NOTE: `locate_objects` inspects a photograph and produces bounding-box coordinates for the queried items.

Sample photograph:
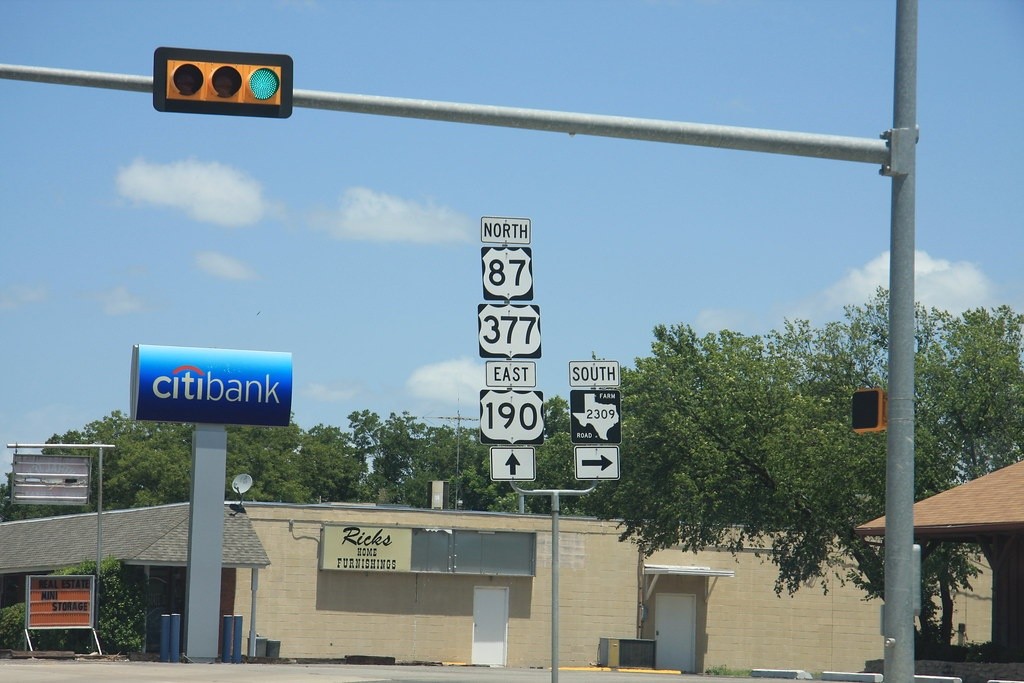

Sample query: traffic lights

[153,47,294,118]
[852,389,882,432]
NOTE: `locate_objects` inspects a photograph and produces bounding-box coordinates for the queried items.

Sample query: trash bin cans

[266,641,281,658]
[247,637,268,657]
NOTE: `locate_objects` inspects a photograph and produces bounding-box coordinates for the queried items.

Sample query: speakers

[852,389,887,436]
